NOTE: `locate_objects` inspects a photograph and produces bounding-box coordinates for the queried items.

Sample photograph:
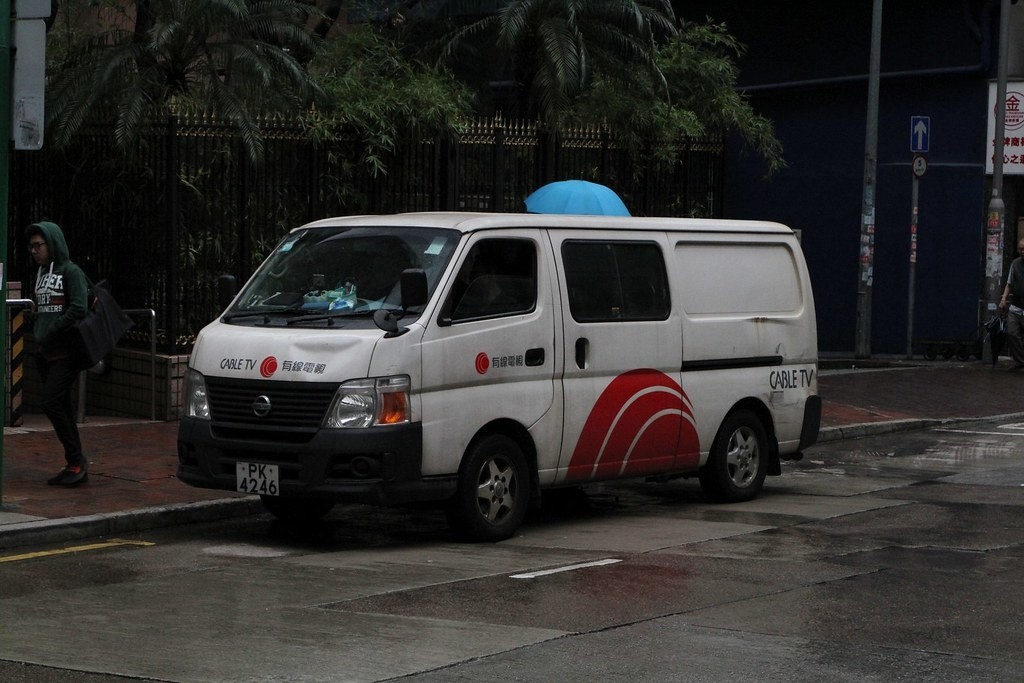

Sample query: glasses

[28,241,45,249]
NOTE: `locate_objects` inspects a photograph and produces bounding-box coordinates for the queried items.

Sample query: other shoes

[47,459,89,488]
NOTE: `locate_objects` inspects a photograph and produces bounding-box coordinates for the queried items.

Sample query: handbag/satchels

[63,263,135,368]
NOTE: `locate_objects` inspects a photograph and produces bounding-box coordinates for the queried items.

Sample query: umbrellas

[983,314,1006,335]
[525,178,632,217]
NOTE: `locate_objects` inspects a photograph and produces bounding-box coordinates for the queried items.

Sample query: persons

[998,239,1024,373]
[22,221,89,487]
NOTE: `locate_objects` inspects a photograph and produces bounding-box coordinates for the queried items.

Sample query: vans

[176,210,821,542]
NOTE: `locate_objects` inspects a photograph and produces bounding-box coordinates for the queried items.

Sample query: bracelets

[1001,299,1006,301]
